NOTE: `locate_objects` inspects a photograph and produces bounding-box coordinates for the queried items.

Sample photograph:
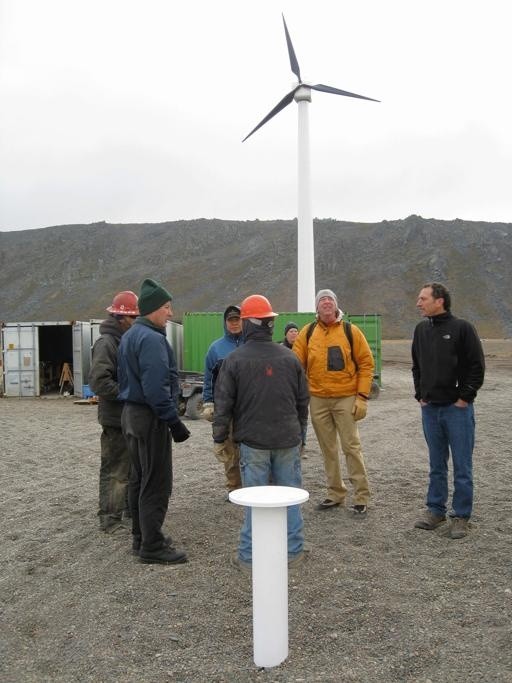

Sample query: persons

[115,279,191,565]
[411,282,485,539]
[212,295,310,574]
[276,322,309,458]
[88,290,141,537]
[201,305,244,503]
[292,289,375,519]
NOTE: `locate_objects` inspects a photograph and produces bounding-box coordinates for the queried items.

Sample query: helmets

[104,289,142,316]
[238,294,280,320]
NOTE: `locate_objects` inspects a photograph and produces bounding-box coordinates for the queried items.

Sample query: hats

[283,320,299,336]
[138,276,174,316]
[224,304,241,320]
[314,287,339,313]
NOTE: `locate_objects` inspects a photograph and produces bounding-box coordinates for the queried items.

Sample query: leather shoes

[224,489,236,503]
[138,541,189,566]
[131,531,174,557]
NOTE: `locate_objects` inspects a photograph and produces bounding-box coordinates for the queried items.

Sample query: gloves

[212,441,231,463]
[202,401,215,422]
[169,419,192,443]
[351,393,370,422]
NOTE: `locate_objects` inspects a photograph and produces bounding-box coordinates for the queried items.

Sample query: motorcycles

[175,370,205,420]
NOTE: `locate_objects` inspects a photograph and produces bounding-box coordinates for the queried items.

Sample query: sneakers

[314,496,344,511]
[352,502,368,520]
[228,548,253,575]
[412,506,448,531]
[286,542,314,570]
[447,514,471,539]
[103,521,132,536]
